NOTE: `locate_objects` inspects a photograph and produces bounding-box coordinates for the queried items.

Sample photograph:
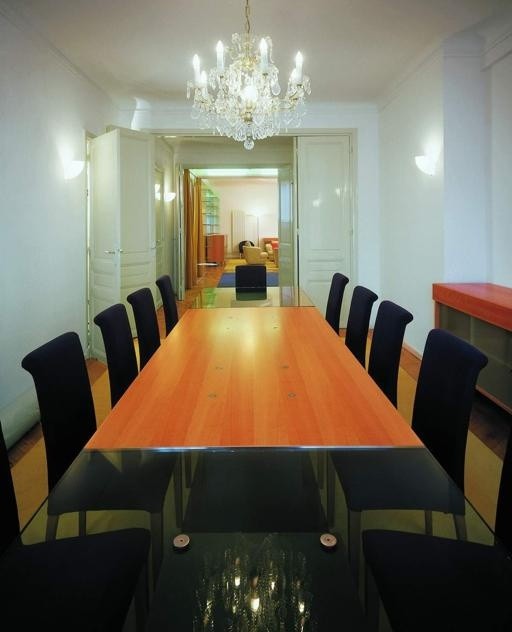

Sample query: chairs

[239,237,279,265]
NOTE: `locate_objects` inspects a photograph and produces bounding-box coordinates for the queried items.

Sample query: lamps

[178,0,313,152]
